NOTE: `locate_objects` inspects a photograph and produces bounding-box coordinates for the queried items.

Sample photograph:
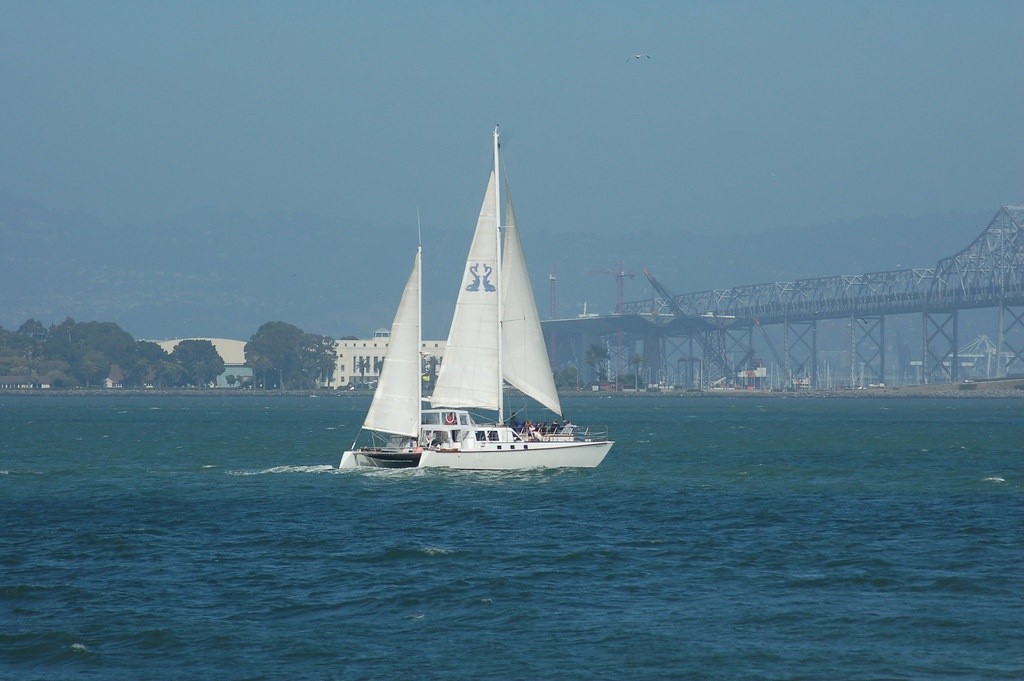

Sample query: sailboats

[337,121,617,473]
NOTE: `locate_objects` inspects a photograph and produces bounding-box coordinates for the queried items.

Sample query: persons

[510,413,569,433]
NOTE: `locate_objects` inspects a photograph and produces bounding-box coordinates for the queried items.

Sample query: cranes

[637,266,738,390]
[548,262,558,319]
[587,260,636,314]
